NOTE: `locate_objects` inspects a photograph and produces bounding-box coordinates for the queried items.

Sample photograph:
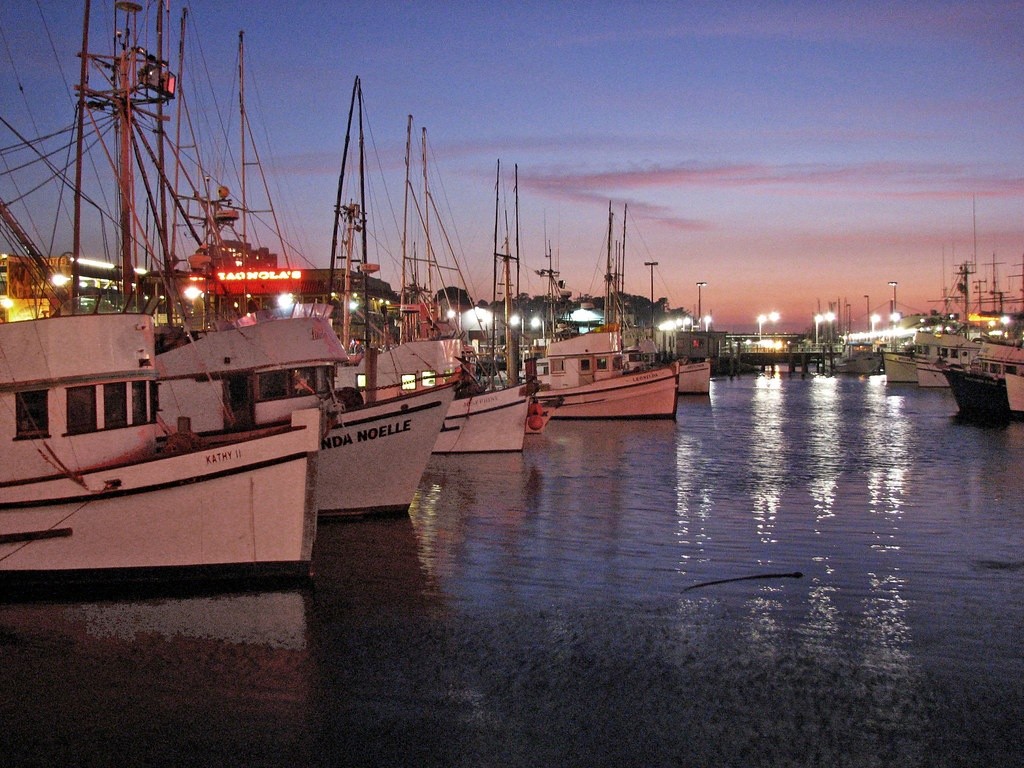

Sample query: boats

[1,1,710,571]
[834,195,1024,417]
